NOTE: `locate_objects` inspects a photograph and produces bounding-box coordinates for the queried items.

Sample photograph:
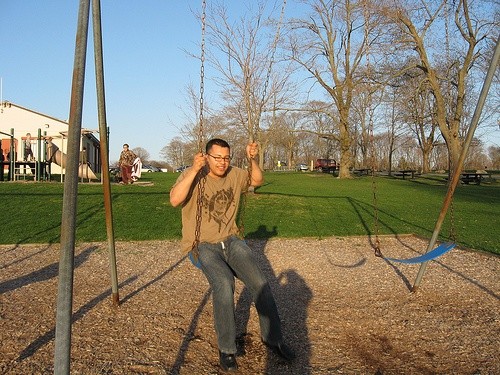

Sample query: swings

[362,0,457,266]
[189,0,287,269]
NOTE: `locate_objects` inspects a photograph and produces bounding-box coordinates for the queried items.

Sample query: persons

[117,144,139,184]
[171,139,295,371]
[24,133,35,162]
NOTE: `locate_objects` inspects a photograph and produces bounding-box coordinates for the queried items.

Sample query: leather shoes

[262,338,295,361]
[218,349,237,368]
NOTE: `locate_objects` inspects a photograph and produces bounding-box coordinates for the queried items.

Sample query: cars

[276,161,287,167]
[141,164,161,173]
[295,163,309,172]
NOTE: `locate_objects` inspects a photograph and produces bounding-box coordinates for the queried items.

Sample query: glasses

[206,153,232,161]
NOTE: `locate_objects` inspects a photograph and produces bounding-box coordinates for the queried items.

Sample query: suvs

[314,158,340,173]
